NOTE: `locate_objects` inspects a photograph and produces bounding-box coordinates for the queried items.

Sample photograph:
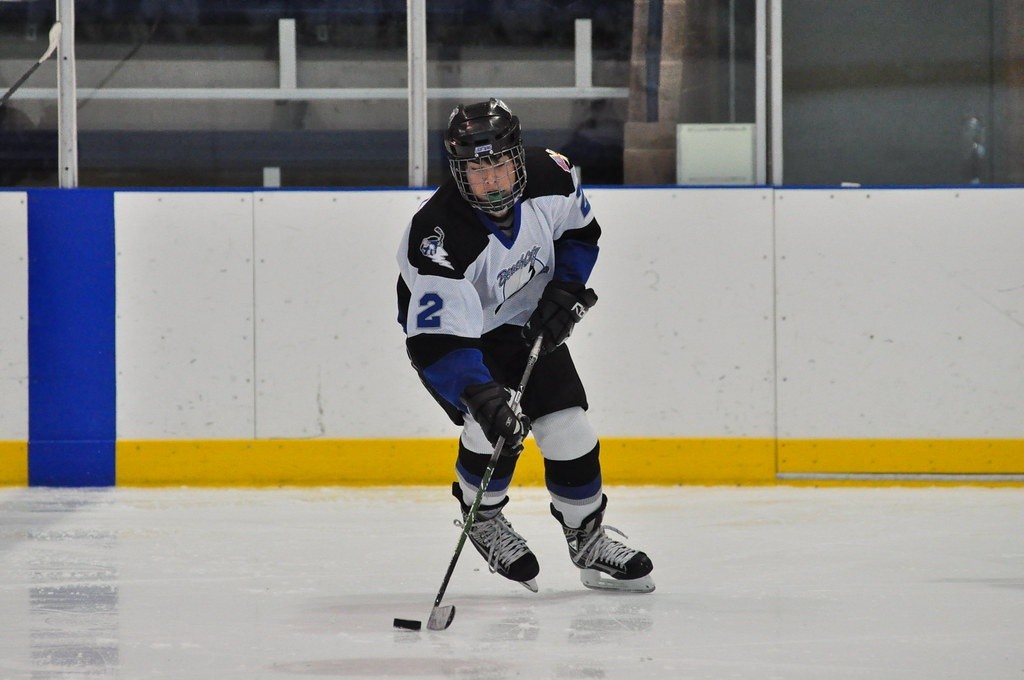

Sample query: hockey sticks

[426,335,546,632]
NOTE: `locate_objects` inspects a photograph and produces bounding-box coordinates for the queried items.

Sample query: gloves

[521,279,598,356]
[459,382,532,457]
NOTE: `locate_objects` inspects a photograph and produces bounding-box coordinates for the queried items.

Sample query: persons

[394,96,661,595]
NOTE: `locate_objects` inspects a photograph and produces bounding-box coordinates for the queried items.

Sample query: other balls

[393,617,421,630]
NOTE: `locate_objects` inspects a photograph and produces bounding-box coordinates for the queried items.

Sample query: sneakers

[452,482,540,593]
[550,494,656,593]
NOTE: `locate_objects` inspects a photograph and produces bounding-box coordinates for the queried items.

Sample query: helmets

[444,97,527,213]
